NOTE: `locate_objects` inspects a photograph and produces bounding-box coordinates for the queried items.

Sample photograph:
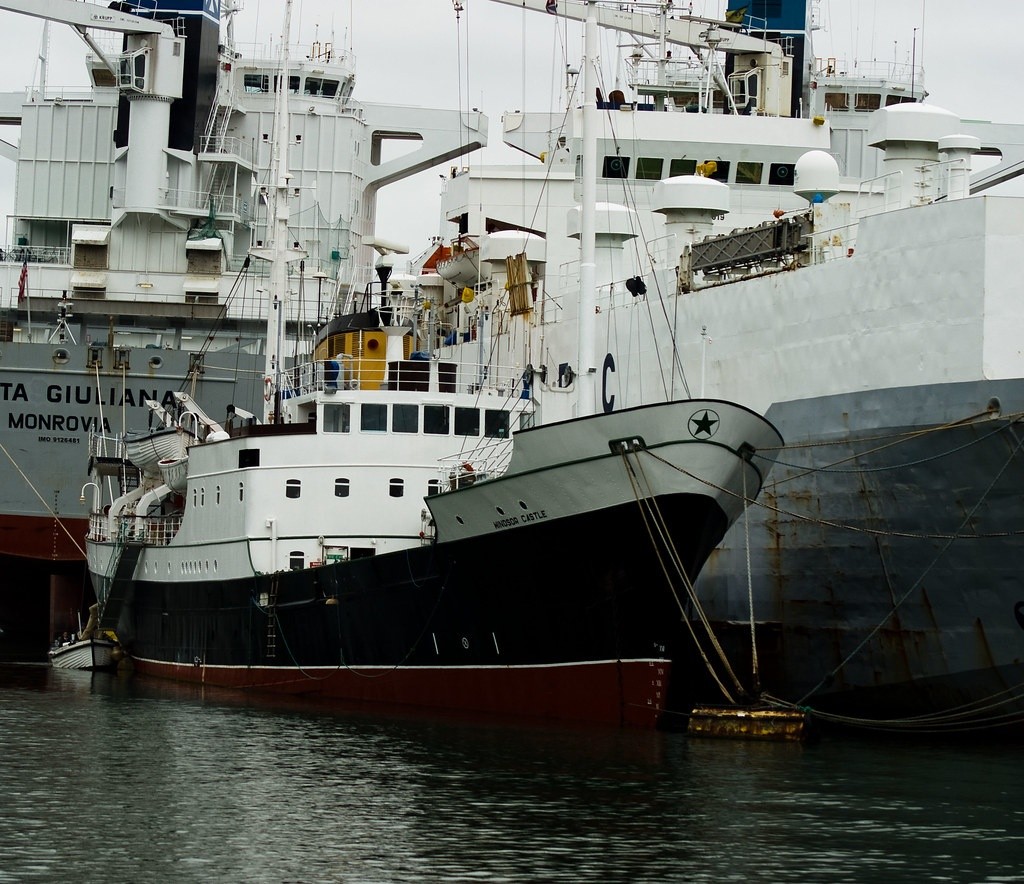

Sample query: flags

[18,252,28,303]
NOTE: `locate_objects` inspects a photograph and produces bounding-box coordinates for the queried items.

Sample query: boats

[2,1,1023,741]
[49,637,112,671]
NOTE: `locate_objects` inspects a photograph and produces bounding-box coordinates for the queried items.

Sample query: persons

[59,631,71,648]
